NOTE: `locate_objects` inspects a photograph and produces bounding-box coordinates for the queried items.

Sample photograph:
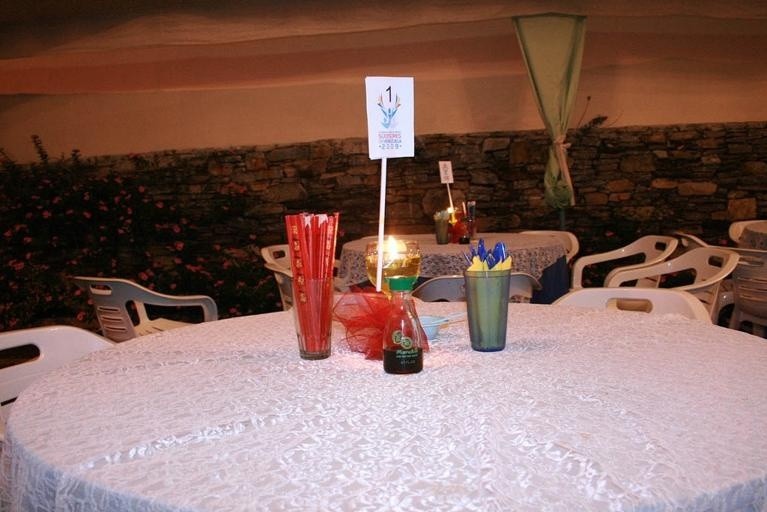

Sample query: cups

[289,276,335,361]
[463,268,512,353]
[435,222,449,244]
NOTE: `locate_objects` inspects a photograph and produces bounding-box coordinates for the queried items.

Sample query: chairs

[0,277,217,436]
[259,220,765,338]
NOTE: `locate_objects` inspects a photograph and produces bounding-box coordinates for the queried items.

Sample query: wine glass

[363,240,422,300]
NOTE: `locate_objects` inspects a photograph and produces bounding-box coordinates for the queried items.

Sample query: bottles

[382,276,423,375]
